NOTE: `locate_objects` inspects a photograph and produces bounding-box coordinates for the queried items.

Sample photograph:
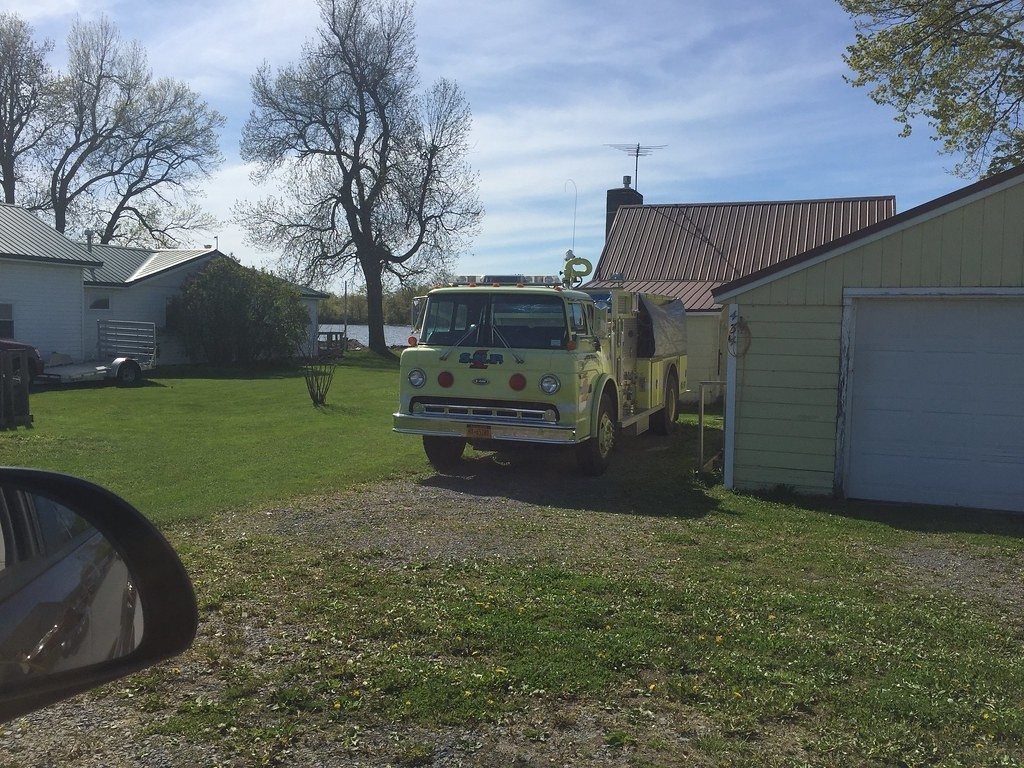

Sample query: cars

[0,340,44,392]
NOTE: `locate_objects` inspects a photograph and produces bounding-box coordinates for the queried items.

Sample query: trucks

[393,257,687,477]
